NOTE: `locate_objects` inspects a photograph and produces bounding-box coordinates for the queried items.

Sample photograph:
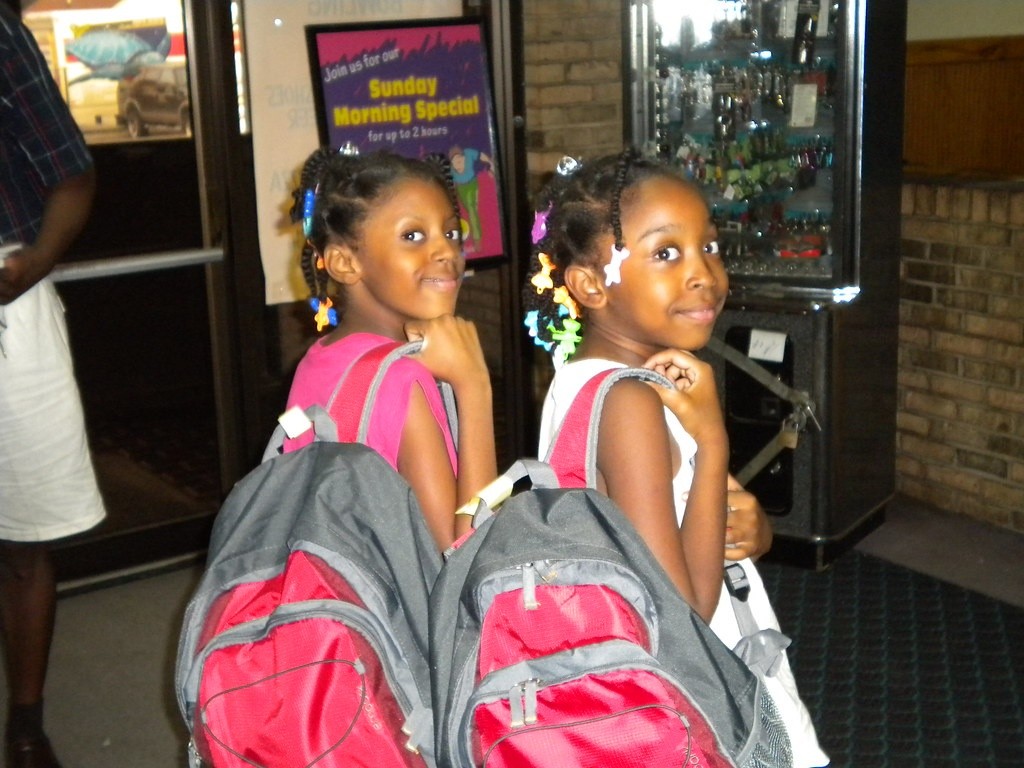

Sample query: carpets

[754,548,1024,768]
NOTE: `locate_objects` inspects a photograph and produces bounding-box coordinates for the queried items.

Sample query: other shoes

[4,728,61,768]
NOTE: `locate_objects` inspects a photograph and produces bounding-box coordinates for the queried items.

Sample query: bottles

[646,1,841,271]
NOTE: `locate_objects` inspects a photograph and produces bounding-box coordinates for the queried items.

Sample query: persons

[0,14,113,767]
[522,152,830,768]
[284,142,494,563]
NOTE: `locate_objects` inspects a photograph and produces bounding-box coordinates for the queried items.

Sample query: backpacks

[431,369,808,768]
[172,340,458,768]
